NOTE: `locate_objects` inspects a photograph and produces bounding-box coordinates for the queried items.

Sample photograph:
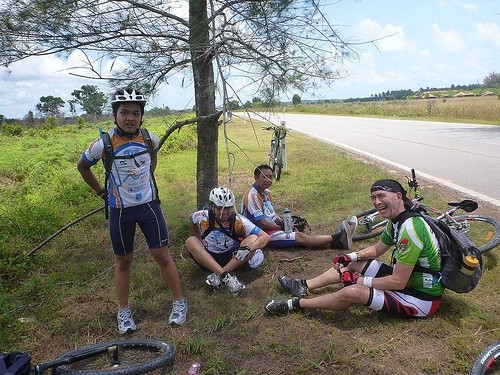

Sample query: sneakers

[347,215,358,236]
[265,296,301,317]
[339,221,353,250]
[168,298,189,326]
[222,272,246,296]
[278,275,310,299]
[117,307,137,335]
[206,272,223,291]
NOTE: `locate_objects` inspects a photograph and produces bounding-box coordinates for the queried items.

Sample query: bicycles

[341,168,500,257]
[262,123,292,183]
[1,338,201,375]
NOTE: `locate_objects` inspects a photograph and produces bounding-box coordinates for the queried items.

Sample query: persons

[265,179,445,317]
[240,165,357,250]
[77,89,187,335]
[186,186,268,296]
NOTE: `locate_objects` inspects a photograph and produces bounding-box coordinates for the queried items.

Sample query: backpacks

[281,215,311,234]
[390,208,483,293]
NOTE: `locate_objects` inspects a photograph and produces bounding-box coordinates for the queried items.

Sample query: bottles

[283,208,293,233]
[455,255,479,289]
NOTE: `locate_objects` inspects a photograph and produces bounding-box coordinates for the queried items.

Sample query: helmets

[208,186,235,207]
[111,87,147,115]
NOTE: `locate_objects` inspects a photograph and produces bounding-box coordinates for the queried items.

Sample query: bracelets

[95,188,108,199]
[363,276,373,287]
[346,251,362,262]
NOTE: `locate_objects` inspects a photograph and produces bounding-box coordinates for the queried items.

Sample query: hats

[372,179,407,199]
